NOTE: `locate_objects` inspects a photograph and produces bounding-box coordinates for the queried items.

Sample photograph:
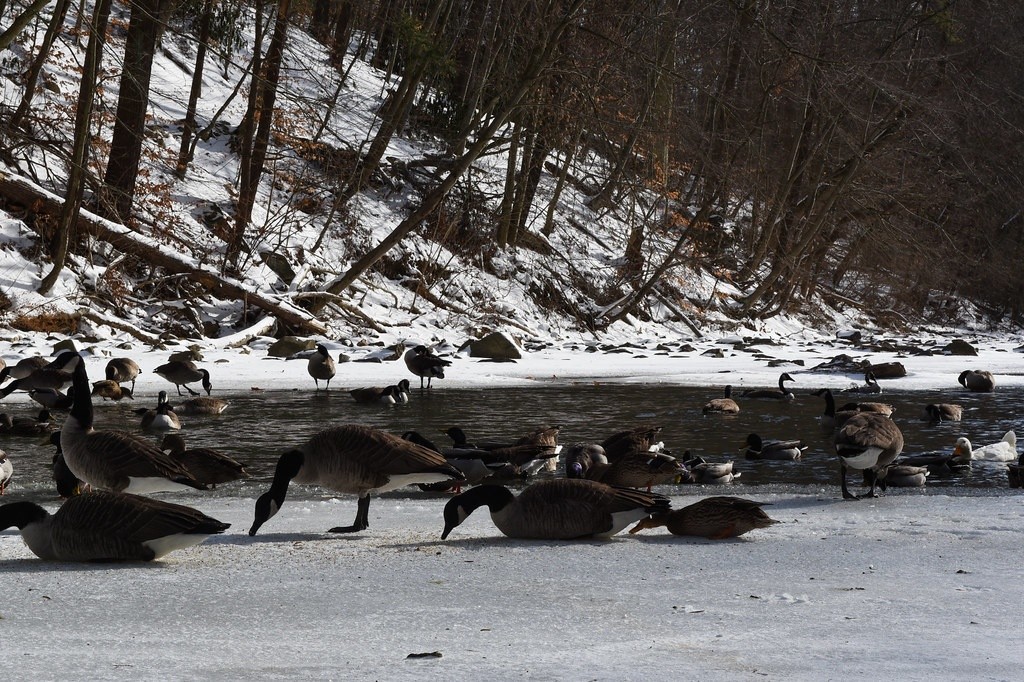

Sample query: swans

[953,429,1018,462]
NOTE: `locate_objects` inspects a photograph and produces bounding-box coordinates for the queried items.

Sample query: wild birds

[0,343,963,567]
[958,370,995,391]
